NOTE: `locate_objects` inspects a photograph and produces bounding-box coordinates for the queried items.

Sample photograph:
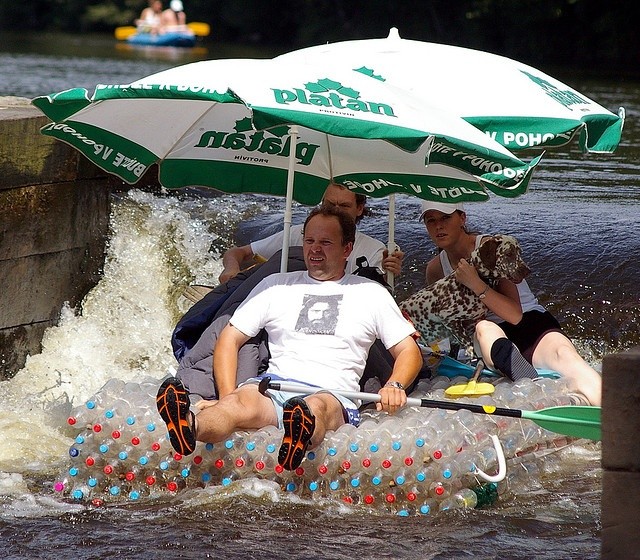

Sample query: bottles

[80,364,584,517]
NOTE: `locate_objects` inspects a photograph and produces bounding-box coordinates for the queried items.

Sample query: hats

[419,199,466,222]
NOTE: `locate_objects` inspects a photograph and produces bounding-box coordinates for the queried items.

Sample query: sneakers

[491,338,539,384]
[156,377,196,456]
[278,396,315,471]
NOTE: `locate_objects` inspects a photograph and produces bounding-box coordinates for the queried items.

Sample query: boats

[126,26,198,45]
[115,23,211,40]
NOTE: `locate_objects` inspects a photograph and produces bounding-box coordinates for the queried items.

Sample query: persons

[133,0,162,34]
[157,203,423,472]
[160,0,186,34]
[418,200,602,408]
[219,182,404,284]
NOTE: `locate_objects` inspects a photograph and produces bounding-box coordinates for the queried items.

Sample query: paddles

[258,377,601,439]
[446,357,494,396]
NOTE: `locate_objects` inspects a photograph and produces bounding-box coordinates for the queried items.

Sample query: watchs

[479,286,491,300]
[383,381,405,390]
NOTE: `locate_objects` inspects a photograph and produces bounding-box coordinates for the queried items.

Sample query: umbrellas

[271,27,620,295]
[29,58,546,273]
